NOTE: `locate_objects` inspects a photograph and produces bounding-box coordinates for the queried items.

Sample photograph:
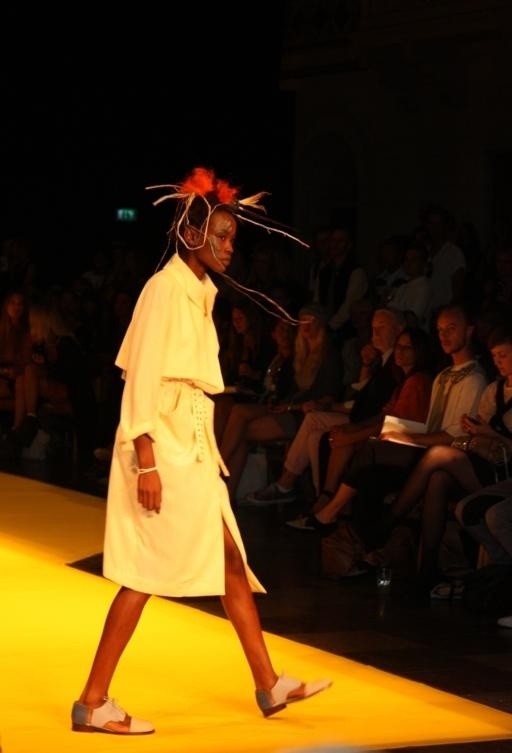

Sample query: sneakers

[247,482,295,504]
[285,515,338,533]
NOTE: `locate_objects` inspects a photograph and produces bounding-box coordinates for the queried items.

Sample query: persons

[67,188,337,738]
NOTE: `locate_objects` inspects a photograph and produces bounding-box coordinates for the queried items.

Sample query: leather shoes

[255,677,333,718]
[72,699,155,735]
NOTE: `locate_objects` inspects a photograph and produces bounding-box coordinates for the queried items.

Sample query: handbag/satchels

[451,435,510,466]
